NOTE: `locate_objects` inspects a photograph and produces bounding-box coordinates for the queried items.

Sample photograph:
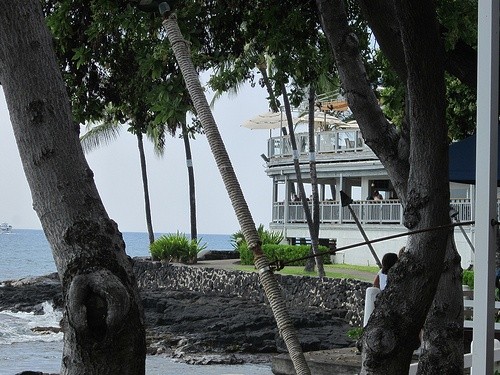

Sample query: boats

[0,223,13,232]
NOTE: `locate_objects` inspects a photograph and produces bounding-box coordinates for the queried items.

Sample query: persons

[373,189,383,200]
[372,254,399,291]
[292,195,314,202]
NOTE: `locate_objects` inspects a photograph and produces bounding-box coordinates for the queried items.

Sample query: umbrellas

[449,136,476,266]
[240,110,359,156]
